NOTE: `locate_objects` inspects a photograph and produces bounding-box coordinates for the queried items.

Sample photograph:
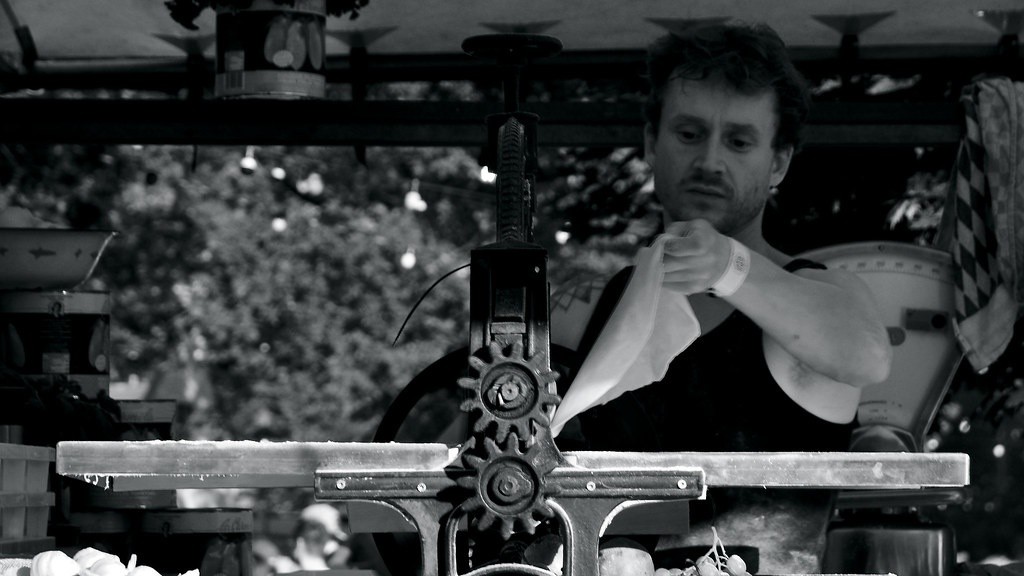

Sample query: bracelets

[712,237,750,296]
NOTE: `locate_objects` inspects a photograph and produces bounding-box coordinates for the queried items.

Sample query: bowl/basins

[0,226,122,293]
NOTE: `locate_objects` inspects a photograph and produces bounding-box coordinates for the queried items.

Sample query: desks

[56,440,971,576]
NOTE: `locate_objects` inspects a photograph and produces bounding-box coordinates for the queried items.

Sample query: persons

[550,23,892,576]
